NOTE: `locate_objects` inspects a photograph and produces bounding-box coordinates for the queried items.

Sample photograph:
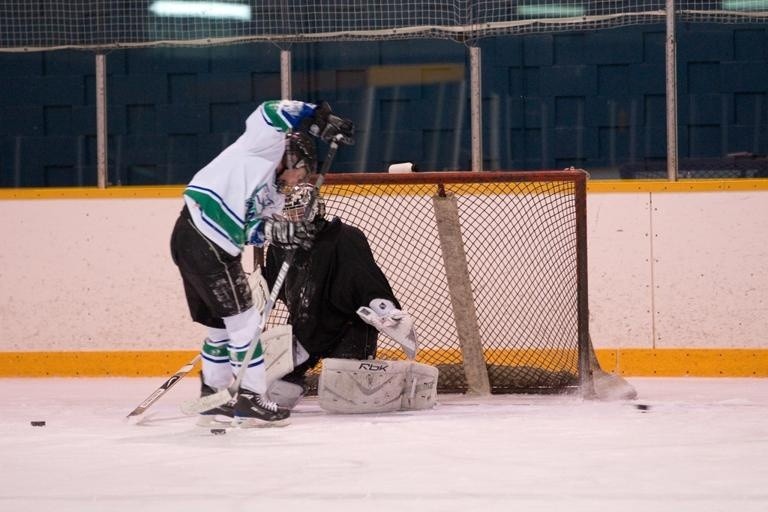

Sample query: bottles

[388,162,418,174]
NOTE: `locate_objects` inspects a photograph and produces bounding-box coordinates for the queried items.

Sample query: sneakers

[200,371,290,420]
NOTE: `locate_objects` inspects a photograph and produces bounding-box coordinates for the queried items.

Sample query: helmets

[274,132,317,196]
[282,183,326,223]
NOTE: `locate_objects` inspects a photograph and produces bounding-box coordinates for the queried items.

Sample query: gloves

[271,219,310,251]
[300,102,356,147]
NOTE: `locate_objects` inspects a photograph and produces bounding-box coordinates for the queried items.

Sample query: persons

[249,183,439,414]
[170,99,355,427]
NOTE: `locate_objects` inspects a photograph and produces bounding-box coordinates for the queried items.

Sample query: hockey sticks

[180,132,343,417]
